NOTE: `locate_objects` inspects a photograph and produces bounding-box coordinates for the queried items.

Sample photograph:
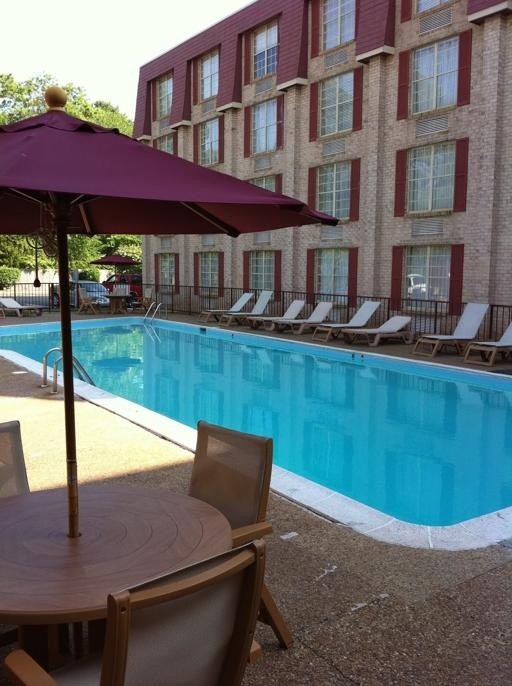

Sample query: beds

[0,297,49,319]
[198,293,254,324]
[311,300,381,343]
[462,318,511,372]
[217,289,274,327]
[246,300,305,332]
[411,301,489,359]
[340,314,414,349]
[272,302,334,335]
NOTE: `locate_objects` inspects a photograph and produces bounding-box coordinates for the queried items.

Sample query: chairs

[187,421,297,657]
[2,540,268,686]
[77,288,99,315]
[130,286,153,314]
[1,419,33,499]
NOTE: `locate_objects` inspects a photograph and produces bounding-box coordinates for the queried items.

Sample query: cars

[102,274,143,297]
[53,281,109,307]
[408,272,432,299]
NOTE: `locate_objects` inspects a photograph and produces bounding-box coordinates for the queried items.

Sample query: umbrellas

[1,84,340,535]
[89,255,141,285]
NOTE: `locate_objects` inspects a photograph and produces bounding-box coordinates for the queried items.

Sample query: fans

[25,210,60,287]
